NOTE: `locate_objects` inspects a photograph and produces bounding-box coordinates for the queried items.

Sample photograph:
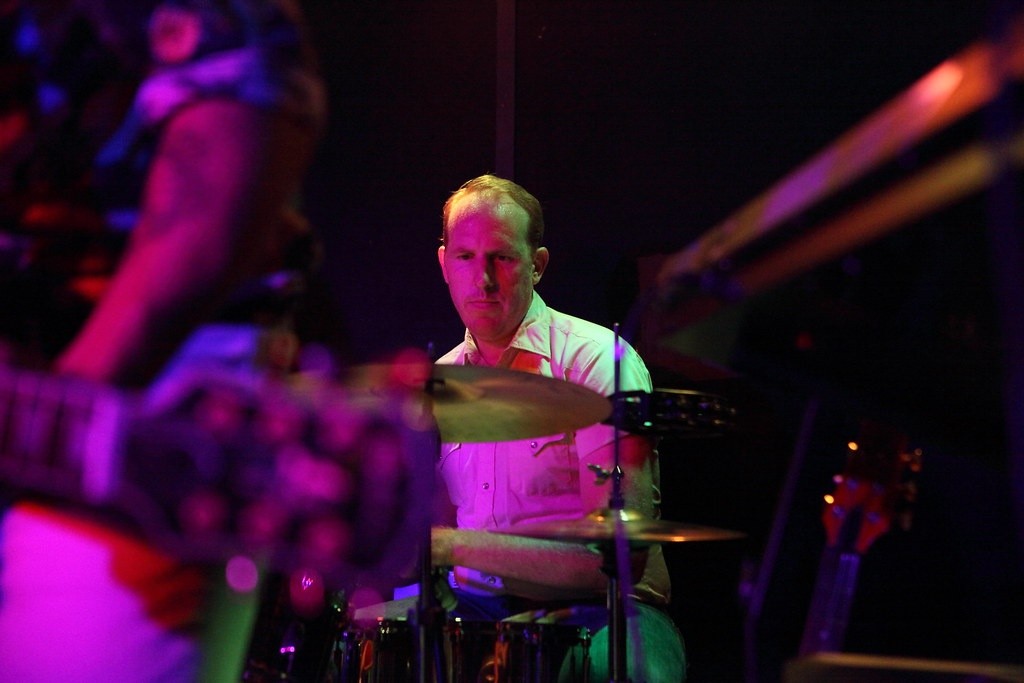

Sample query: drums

[340,615,590,683]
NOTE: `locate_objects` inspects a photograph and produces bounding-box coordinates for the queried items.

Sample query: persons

[133,0,321,397]
[422,175,688,682]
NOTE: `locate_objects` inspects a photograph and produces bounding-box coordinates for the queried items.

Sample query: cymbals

[275,361,614,445]
[597,386,754,441]
[485,507,750,544]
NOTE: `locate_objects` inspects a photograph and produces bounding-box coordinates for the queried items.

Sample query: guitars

[799,474,891,654]
